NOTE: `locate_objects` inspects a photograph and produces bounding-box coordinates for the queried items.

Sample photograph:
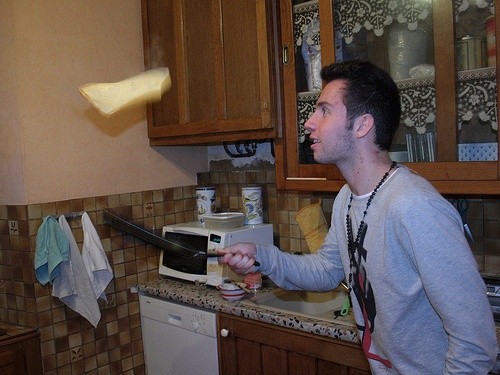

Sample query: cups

[473,251,500,275]
[195,186,216,225]
[241,187,262,225]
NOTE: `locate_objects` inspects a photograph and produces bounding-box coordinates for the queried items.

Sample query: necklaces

[345,161,398,291]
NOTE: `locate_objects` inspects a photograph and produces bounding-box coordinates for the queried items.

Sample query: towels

[33,215,70,286]
[81,211,114,305]
[50,214,102,328]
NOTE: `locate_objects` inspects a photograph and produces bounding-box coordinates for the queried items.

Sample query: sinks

[240,291,346,317]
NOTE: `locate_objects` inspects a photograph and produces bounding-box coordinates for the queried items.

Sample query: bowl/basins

[388,145,409,164]
[220,283,248,300]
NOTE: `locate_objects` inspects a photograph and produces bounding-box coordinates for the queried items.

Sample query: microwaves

[159,222,274,286]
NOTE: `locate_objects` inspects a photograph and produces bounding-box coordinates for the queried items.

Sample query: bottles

[455,14,497,70]
[243,271,262,290]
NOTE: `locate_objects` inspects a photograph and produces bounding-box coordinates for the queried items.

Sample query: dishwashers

[139,294,219,375]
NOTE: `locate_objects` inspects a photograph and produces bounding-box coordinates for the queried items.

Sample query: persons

[216,62,499,375]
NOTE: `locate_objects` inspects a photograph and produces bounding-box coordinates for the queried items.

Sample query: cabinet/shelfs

[220,318,372,375]
[275,0,499,190]
[141,0,276,138]
